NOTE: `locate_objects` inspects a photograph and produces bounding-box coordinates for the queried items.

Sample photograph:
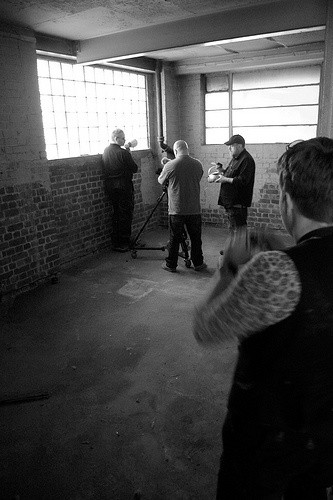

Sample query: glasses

[284,139,304,189]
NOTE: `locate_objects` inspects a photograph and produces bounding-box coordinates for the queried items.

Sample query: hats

[224,134,245,145]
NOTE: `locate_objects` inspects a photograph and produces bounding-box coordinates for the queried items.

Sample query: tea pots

[207,166,224,182]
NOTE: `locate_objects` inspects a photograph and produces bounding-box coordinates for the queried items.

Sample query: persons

[101,129,138,251]
[158,139,207,271]
[193,136,333,500]
[212,134,255,255]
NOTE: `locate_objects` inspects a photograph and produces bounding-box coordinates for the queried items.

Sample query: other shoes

[195,263,206,270]
[112,246,128,252]
[162,263,176,272]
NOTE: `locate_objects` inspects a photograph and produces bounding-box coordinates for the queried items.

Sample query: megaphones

[125,138,137,149]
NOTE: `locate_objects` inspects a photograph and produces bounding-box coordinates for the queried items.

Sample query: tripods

[135,182,187,253]
